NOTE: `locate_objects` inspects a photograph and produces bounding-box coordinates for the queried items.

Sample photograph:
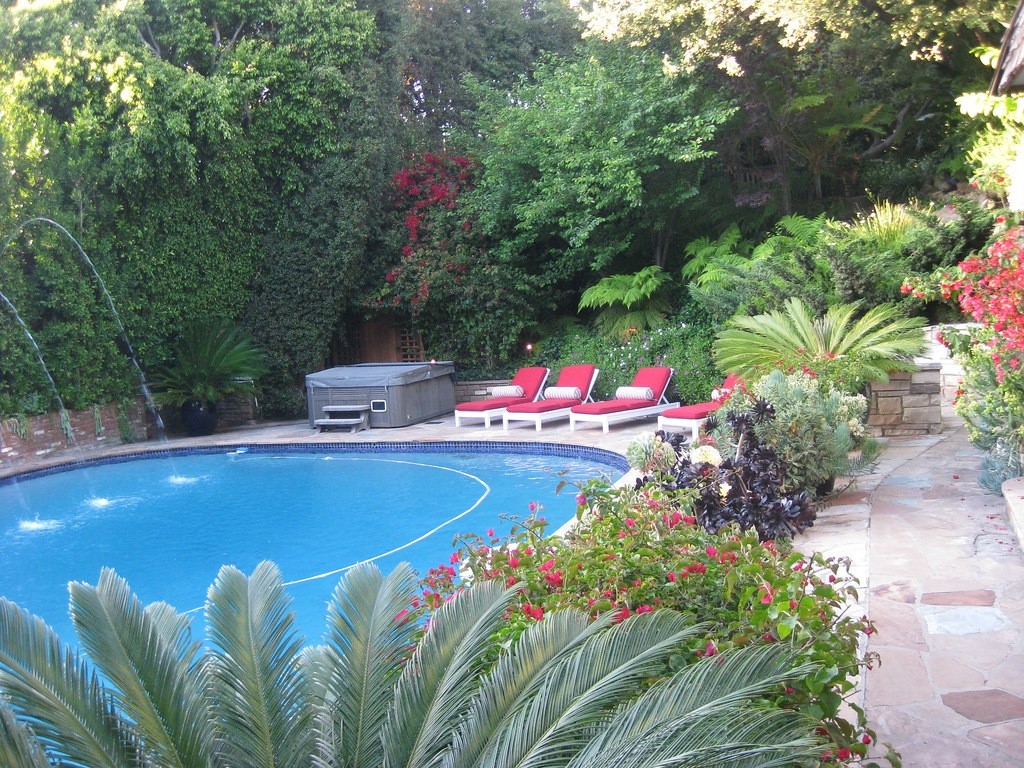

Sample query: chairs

[503,364,599,432]
[454,366,550,427]
[570,366,681,434]
[658,374,751,439]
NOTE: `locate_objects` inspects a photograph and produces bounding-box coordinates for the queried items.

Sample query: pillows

[492,385,524,397]
[616,386,653,401]
[545,387,582,400]
[711,387,730,400]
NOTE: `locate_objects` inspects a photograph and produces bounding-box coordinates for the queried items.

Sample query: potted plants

[147,313,276,438]
[711,297,931,494]
